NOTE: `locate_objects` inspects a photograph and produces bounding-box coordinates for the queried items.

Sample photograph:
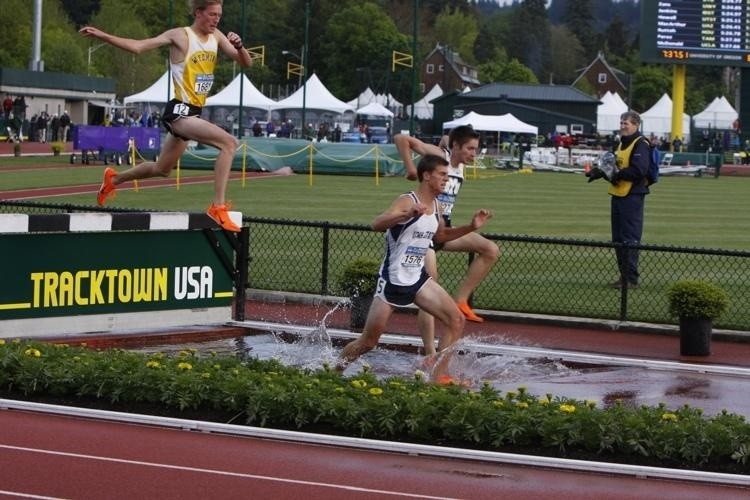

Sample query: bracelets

[234,44,242,49]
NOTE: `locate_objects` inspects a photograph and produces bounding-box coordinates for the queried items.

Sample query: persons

[105,111,162,127]
[0,92,72,145]
[651,119,750,178]
[78,0,252,233]
[500,131,619,151]
[585,112,651,289]
[413,126,425,138]
[394,125,499,368]
[337,153,492,387]
[234,117,391,143]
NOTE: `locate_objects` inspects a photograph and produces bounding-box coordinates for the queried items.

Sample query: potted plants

[337,256,386,334]
[14,144,21,157]
[51,143,63,156]
[669,278,732,358]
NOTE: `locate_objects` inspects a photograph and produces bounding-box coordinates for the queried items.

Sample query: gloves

[586,168,601,183]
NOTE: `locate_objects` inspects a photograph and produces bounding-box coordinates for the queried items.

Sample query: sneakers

[457,302,483,324]
[97,167,118,206]
[207,201,241,232]
[435,374,468,387]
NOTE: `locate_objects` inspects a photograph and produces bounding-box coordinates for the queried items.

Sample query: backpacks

[631,136,661,186]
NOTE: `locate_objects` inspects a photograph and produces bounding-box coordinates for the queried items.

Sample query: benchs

[656,149,721,180]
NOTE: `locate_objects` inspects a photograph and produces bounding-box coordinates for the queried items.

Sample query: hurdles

[0,210,252,337]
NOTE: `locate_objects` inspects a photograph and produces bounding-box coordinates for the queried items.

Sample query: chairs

[522,146,609,169]
[660,152,675,167]
[473,147,488,169]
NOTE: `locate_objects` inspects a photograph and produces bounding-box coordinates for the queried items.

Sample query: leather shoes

[614,279,639,289]
